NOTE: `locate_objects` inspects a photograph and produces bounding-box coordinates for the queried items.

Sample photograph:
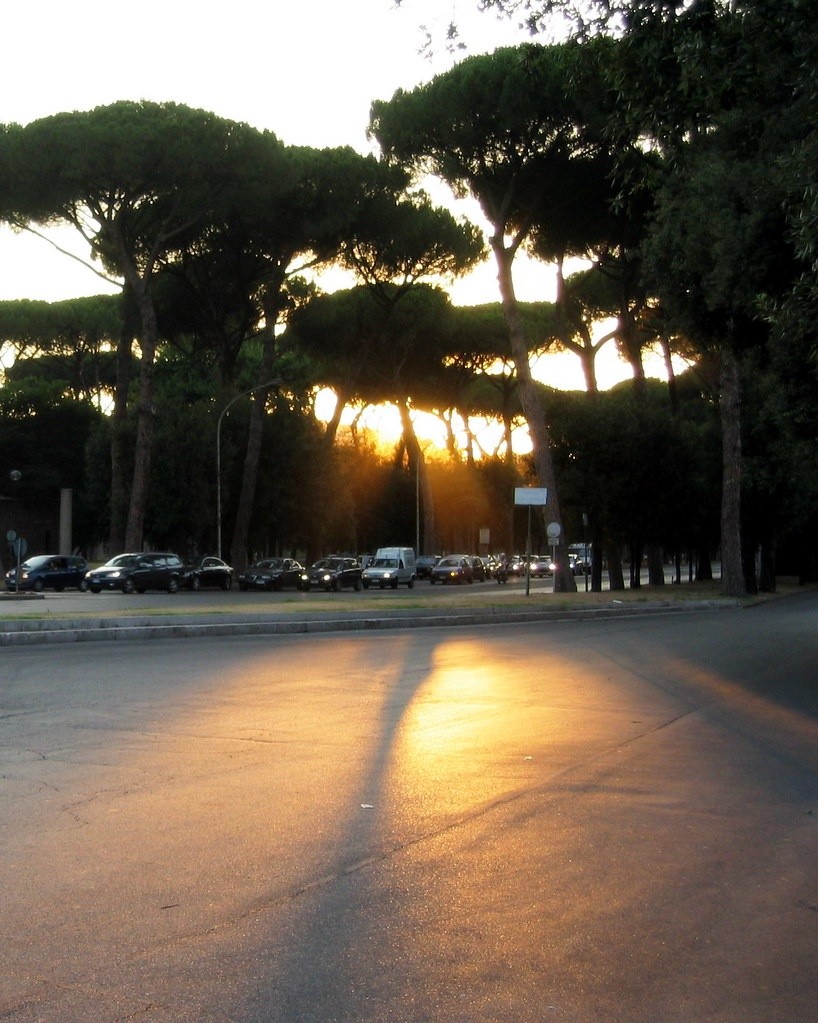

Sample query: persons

[496,552,509,578]
[49,561,58,572]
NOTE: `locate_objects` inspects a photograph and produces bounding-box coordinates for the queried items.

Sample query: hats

[500,552,505,556]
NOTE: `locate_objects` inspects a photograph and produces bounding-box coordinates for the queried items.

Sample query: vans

[360,547,417,590]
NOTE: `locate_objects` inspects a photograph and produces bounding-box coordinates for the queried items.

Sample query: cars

[417,543,593,585]
[178,556,235,592]
[7,554,88,594]
[296,557,363,591]
[84,550,185,596]
[237,557,306,592]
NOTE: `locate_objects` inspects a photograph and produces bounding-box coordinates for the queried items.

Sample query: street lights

[416,435,444,560]
[215,377,286,559]
